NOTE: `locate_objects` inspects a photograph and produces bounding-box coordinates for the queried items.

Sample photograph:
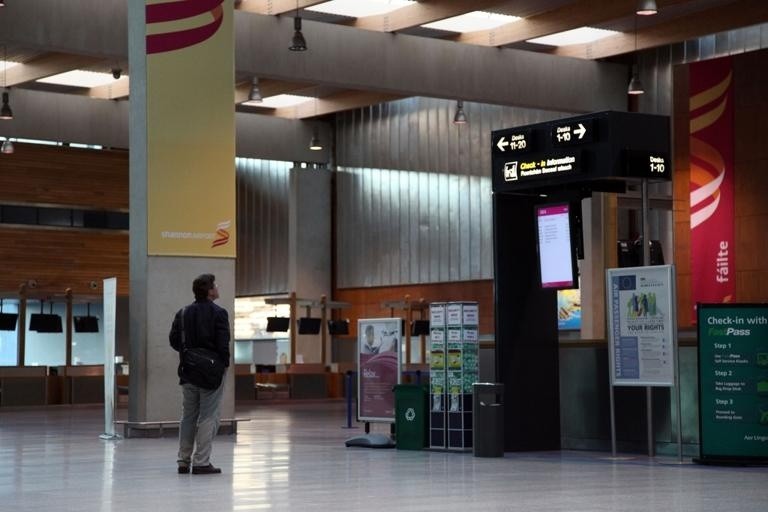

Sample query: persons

[363,326,382,355]
[169,273,230,475]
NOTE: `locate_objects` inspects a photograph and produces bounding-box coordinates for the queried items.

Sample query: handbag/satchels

[177,348,226,390]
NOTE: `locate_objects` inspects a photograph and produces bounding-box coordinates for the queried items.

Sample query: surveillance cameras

[29,280,38,289]
[91,282,97,290]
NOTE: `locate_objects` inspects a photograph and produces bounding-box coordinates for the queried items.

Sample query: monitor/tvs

[266,316,349,336]
[0,314,99,333]
[402,318,430,336]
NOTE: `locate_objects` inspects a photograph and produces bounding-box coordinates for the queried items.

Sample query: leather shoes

[179,465,190,473]
[192,463,223,474]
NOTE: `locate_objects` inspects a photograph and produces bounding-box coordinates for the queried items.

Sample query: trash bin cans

[471,383,504,458]
[392,382,429,450]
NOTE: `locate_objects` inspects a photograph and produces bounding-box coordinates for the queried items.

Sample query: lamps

[453,97,468,125]
[636,0,658,16]
[626,8,646,98]
[1,45,16,154]
[309,123,323,151]
[246,1,308,101]
